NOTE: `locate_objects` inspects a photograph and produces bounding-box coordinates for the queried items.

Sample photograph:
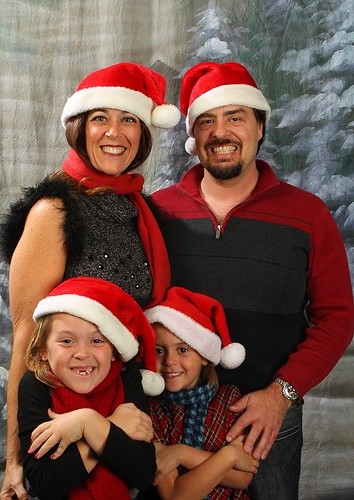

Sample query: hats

[179,62,272,156]
[61,61,181,141]
[32,277,165,397]
[142,286,246,369]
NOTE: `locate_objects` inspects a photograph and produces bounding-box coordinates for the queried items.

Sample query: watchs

[274,377,300,404]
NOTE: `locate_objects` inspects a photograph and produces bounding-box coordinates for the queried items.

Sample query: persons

[147,62,354,500]
[17,277,165,500]
[0,63,182,500]
[144,286,260,500]
